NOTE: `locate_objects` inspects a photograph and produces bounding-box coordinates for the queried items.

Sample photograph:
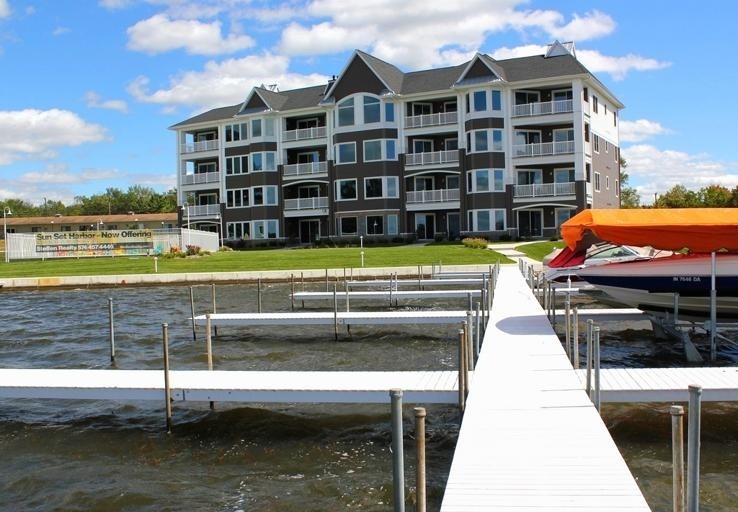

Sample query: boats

[543,207,738,316]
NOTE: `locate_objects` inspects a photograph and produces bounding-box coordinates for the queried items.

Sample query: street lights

[97,218,103,229]
[180,201,190,229]
[214,211,223,247]
[3,206,12,262]
